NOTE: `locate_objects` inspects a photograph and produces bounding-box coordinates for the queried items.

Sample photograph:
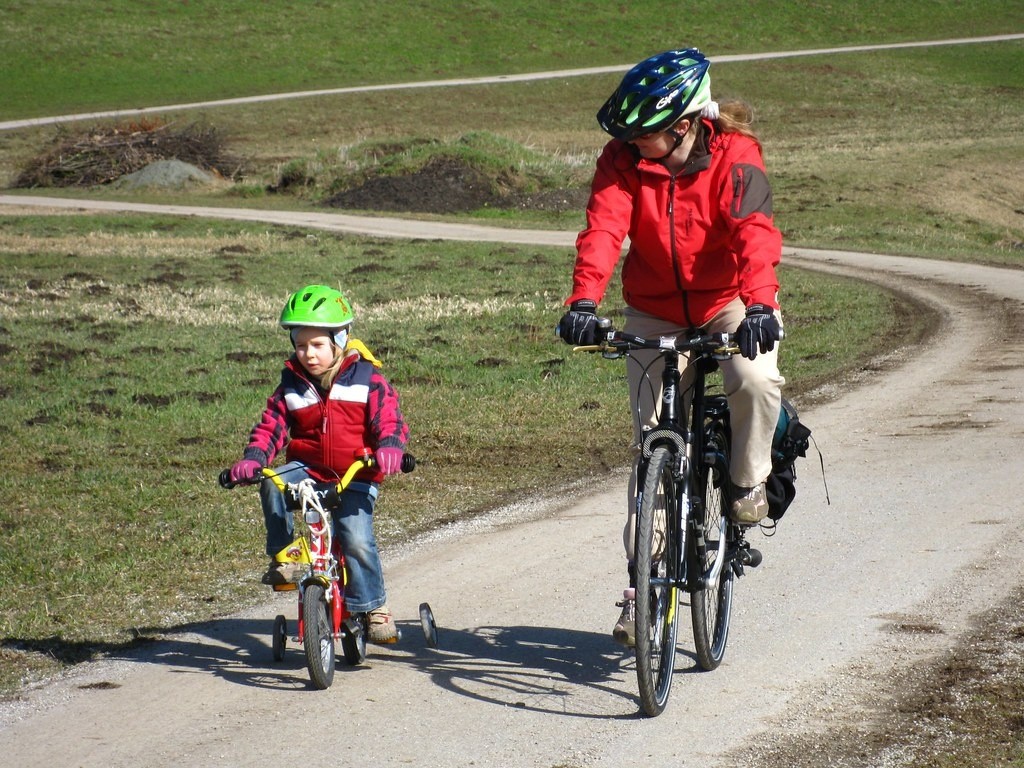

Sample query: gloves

[376,436,406,475]
[230,447,267,487]
[560,299,604,354]
[735,303,780,360]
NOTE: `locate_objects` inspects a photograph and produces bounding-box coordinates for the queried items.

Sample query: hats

[291,327,347,349]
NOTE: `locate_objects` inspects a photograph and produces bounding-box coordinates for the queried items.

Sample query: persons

[232,282,410,645]
[561,48,783,647]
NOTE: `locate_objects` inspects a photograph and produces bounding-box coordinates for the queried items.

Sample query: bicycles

[217,453,440,691]
[554,311,805,718]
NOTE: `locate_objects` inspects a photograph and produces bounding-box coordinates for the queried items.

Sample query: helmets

[596,48,712,140]
[280,285,354,327]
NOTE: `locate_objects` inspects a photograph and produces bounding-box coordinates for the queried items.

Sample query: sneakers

[262,561,300,585]
[368,605,396,642]
[728,482,769,523]
[613,588,657,646]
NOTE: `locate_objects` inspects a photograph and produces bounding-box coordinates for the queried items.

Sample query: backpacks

[766,395,811,520]
